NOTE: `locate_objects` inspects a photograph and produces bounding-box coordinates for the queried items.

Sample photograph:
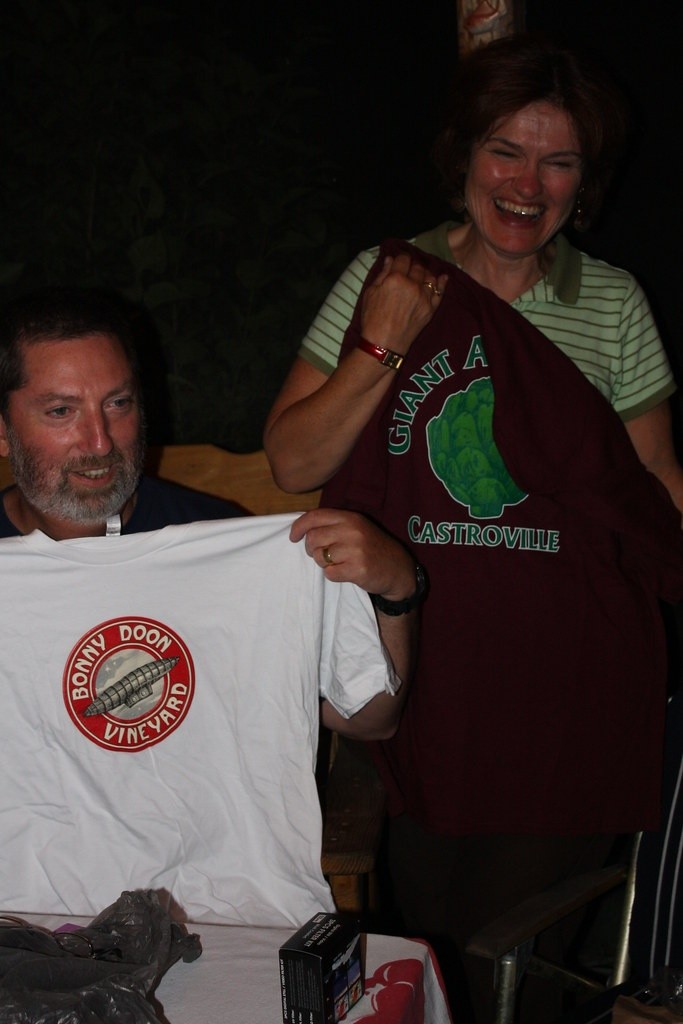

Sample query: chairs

[466,697,683,1024]
[0,444,388,963]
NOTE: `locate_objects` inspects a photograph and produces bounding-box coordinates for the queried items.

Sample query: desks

[0,910,451,1024]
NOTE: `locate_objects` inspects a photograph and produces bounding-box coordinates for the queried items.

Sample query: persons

[264,30,682,1005]
[0,289,418,927]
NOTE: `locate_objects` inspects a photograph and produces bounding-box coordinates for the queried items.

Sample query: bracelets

[372,562,429,617]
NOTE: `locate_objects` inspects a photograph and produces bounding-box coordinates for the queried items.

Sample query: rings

[324,547,332,565]
[424,281,441,297]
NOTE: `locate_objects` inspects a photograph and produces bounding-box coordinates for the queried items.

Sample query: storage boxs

[278,912,365,1024]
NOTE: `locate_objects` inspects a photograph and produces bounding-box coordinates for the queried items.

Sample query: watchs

[357,337,405,371]
